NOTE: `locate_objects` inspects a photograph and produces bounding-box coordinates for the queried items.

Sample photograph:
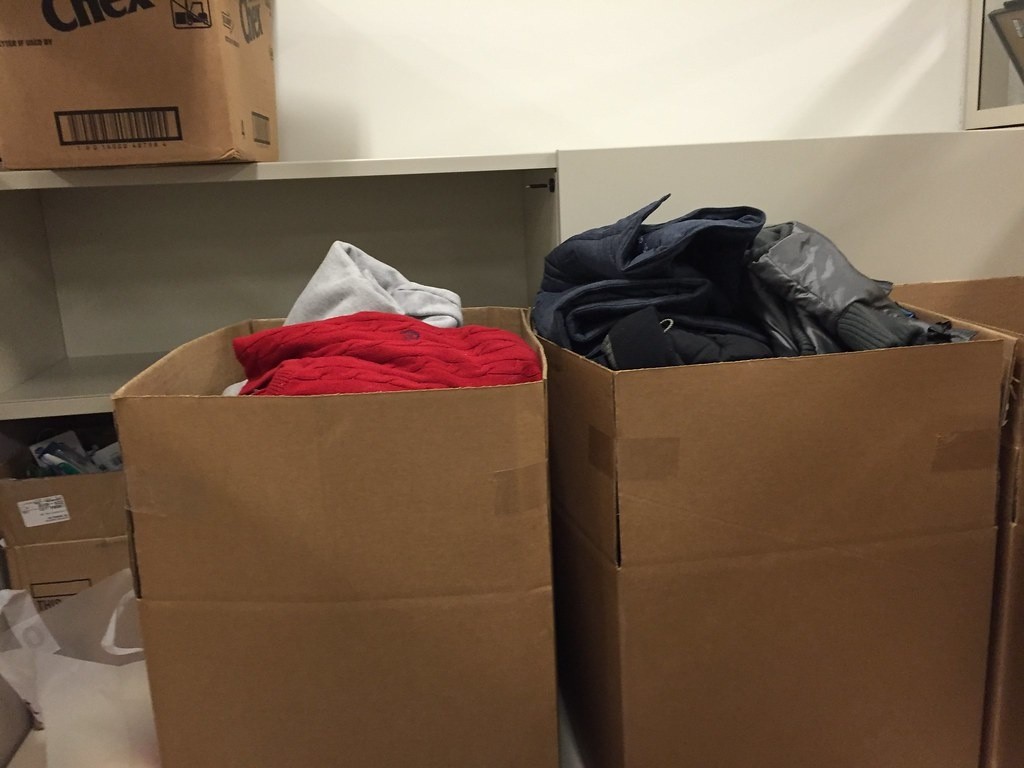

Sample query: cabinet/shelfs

[0,150,564,424]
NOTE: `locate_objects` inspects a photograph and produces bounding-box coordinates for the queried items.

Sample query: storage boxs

[0,450,138,614]
[110,304,561,768]
[888,277,1023,767]
[527,303,1022,767]
[0,0,278,170]
